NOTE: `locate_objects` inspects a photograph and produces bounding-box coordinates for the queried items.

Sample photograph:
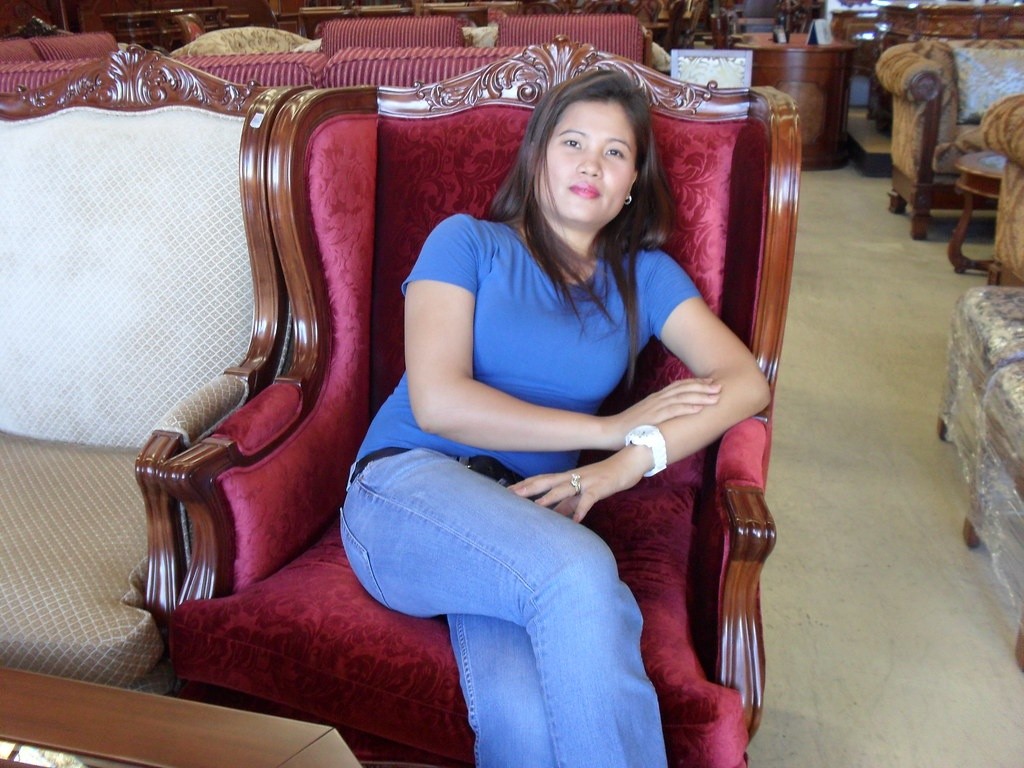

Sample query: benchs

[735,27,859,172]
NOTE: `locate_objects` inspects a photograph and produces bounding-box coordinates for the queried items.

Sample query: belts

[350,447,525,485]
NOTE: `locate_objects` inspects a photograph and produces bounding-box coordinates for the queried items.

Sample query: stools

[941,285,1024,673]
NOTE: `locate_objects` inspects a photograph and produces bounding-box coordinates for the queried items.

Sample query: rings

[570,472,582,494]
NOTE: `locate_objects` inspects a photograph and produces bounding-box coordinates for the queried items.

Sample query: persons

[341,67,773,767]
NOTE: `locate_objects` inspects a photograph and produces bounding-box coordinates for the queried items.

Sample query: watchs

[625,425,667,478]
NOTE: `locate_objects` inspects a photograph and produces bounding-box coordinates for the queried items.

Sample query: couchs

[163,38,801,768]
[312,11,672,77]
[875,36,1024,242]
[980,93,1024,286]
[0,40,313,692]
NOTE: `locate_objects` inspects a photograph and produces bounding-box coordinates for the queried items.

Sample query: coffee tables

[948,154,1008,273]
[0,665,362,768]
